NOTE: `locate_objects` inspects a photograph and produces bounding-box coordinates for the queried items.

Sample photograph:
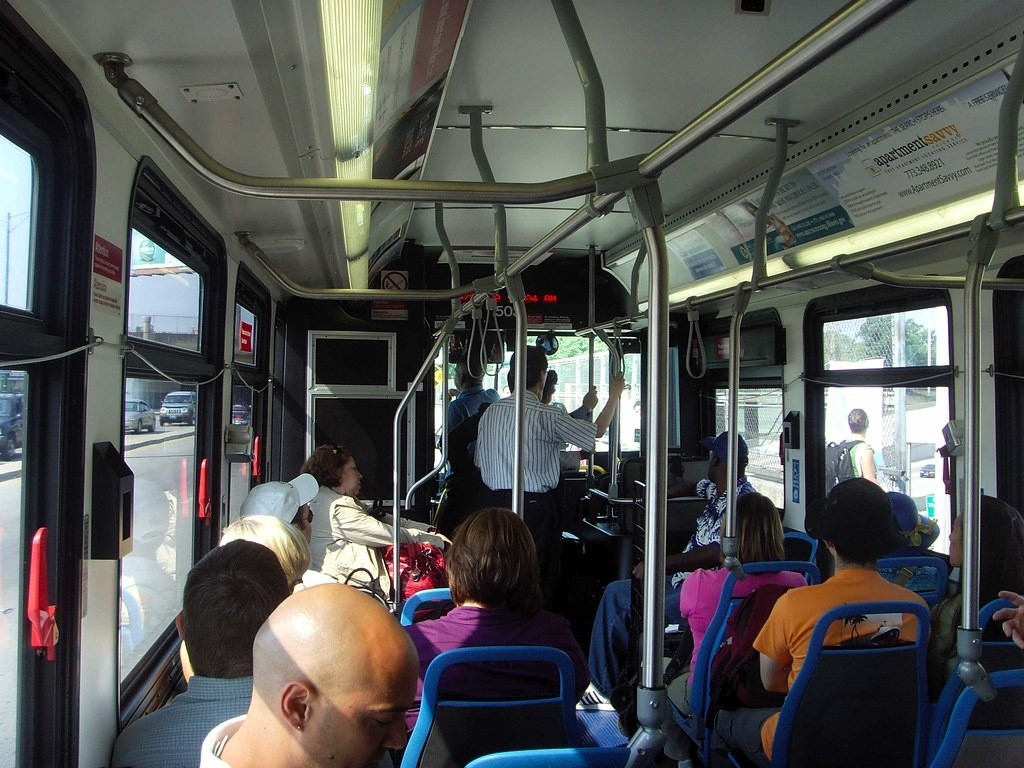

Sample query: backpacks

[825,439,864,495]
[708,584,800,711]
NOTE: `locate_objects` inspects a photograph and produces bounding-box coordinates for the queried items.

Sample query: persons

[300,444,453,601]
[474,346,625,611]
[387,506,590,768]
[575,430,757,712]
[845,409,881,485]
[665,476,1024,768]
[180,472,319,681]
[621,384,631,402]
[437,356,501,472]
[632,399,641,416]
[110,539,419,768]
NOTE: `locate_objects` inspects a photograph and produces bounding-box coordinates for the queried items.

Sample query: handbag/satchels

[382,540,453,622]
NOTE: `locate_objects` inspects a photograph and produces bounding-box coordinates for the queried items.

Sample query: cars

[231,404,249,425]
[919,464,935,478]
[124,399,156,434]
[0,392,23,462]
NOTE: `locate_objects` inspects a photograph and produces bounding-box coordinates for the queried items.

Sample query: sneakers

[575,683,617,712]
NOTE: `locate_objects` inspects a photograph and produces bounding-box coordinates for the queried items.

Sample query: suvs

[158,390,197,426]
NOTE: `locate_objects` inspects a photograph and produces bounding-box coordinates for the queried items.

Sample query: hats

[885,490,941,558]
[701,432,748,467]
[805,477,911,554]
[241,473,319,524]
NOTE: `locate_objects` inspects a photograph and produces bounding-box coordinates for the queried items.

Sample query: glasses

[292,509,314,525]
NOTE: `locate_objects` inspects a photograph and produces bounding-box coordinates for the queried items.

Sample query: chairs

[925,598,1024,768]
[667,532,947,768]
[398,645,582,767]
